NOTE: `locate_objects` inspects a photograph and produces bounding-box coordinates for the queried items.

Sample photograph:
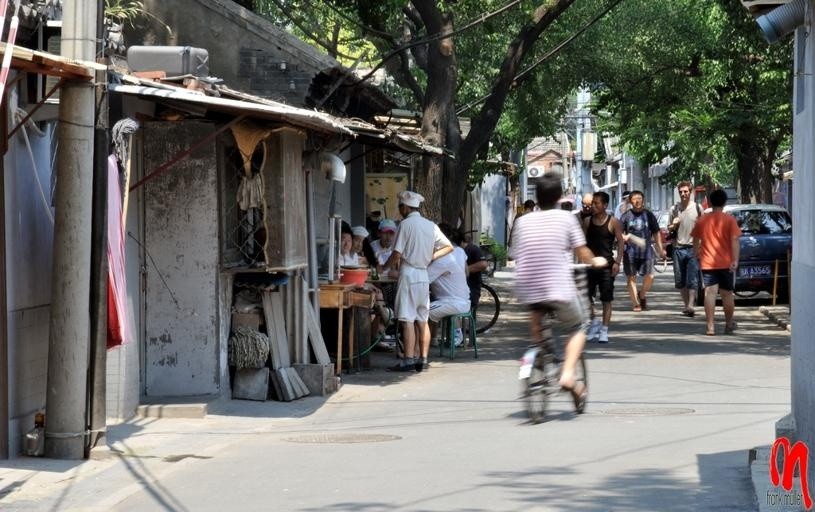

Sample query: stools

[440,312,479,360]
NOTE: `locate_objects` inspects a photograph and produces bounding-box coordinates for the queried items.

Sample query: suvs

[694,204,792,305]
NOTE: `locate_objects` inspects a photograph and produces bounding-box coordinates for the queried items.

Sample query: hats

[379,220,396,232]
[398,191,424,207]
[352,227,369,237]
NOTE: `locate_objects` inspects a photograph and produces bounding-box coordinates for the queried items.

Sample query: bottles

[25,412,46,456]
[371,252,379,280]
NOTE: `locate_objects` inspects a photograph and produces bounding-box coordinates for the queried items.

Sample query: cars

[652,210,673,258]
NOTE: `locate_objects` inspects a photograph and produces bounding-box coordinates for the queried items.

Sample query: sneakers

[454,329,463,347]
[388,362,425,371]
[598,326,608,342]
[638,291,646,309]
[587,320,600,340]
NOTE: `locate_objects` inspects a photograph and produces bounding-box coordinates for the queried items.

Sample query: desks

[367,275,399,358]
[316,282,356,374]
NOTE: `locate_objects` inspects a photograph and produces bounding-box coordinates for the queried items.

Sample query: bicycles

[516,263,596,422]
[651,244,668,274]
[461,284,500,337]
[463,229,496,276]
[734,290,760,298]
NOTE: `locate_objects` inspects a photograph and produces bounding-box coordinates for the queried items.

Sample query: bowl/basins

[339,270,369,287]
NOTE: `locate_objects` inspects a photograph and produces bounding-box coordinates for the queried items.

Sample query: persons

[508,173,609,398]
[690,189,743,335]
[508,190,632,343]
[320,189,486,372]
[619,189,668,311]
[668,182,704,316]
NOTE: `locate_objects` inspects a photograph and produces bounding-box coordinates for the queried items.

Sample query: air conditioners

[528,166,545,178]
